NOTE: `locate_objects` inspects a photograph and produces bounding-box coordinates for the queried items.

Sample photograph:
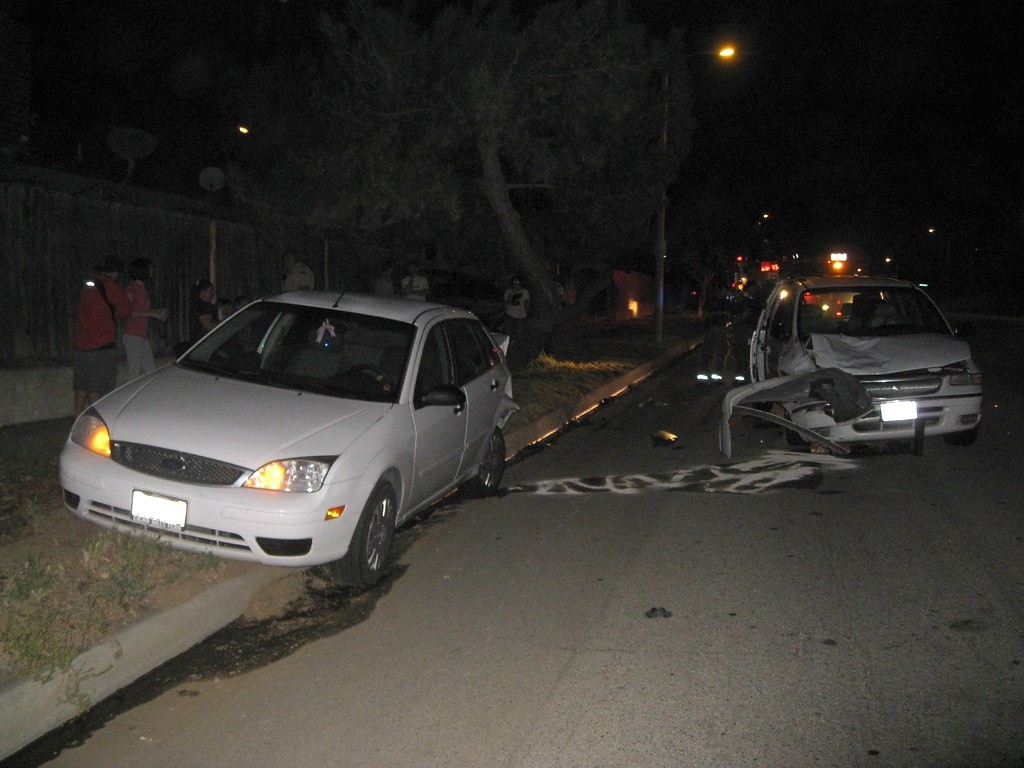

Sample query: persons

[698,269,767,383]
[866,297,904,328]
[402,263,429,301]
[503,276,530,341]
[278,249,315,294]
[71,255,132,419]
[122,257,168,383]
[187,278,266,365]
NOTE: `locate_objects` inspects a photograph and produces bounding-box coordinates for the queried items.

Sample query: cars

[58,287,522,588]
[713,274,986,458]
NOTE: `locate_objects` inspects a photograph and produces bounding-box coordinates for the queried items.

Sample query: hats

[95,255,124,271]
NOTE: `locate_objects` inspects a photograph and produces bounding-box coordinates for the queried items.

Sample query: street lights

[654,42,738,348]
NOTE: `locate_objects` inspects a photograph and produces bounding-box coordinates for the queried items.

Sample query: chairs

[848,295,871,329]
[801,305,827,333]
[371,346,421,398]
[297,323,347,382]
[867,299,898,325]
